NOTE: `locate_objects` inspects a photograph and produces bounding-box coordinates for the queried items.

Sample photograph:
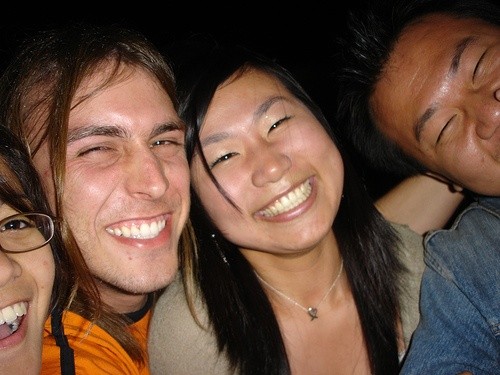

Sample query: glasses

[0,211,64,254]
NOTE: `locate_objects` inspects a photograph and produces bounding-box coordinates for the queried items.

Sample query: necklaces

[249,258,344,323]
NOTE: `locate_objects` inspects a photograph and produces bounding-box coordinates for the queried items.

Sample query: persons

[1,125,75,375]
[331,1,500,375]
[147,47,430,375]
[6,27,464,375]
